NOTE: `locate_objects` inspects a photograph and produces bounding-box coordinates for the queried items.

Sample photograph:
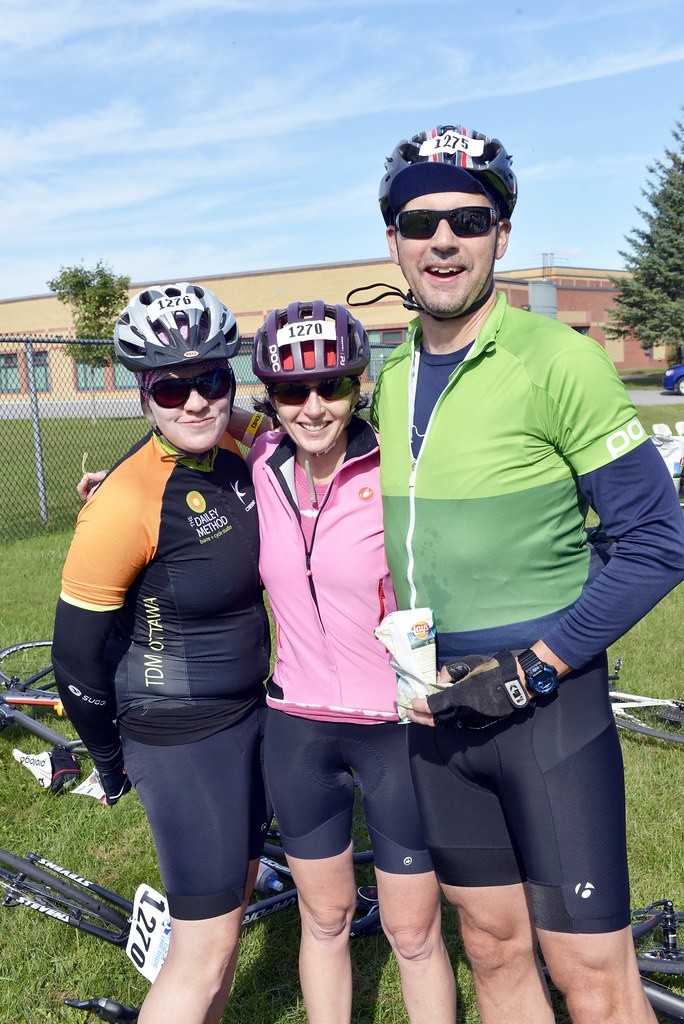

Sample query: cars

[661,363,684,397]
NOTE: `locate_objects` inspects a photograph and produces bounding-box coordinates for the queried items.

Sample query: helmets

[252,301,371,384]
[378,125,518,214]
[115,282,242,372]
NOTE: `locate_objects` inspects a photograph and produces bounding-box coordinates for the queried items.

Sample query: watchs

[517,649,559,702]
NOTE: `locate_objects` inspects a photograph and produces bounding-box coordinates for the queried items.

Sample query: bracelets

[241,411,266,448]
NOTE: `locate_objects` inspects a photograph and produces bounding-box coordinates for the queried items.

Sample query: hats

[389,162,500,216]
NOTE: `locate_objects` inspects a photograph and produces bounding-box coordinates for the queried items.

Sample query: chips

[82,452,88,474]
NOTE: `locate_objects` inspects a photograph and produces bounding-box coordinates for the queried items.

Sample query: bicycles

[1,642,93,795]
[0,824,380,1024]
[521,891,684,1024]
[605,658,684,744]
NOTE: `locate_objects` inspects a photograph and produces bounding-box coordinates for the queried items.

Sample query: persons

[229,124,684,1024]
[76,300,458,1024]
[51,280,273,1024]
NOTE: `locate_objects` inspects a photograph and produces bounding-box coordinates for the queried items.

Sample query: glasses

[141,363,233,409]
[395,206,501,239]
[269,375,355,405]
